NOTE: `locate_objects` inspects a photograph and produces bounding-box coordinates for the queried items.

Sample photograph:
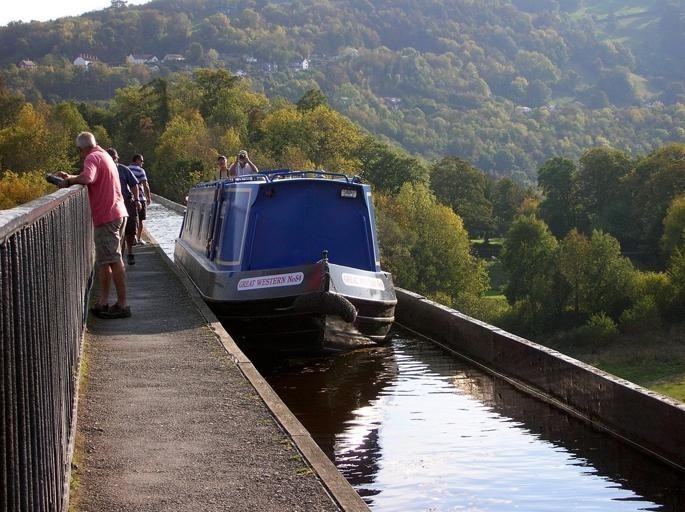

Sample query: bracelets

[134,198,140,203]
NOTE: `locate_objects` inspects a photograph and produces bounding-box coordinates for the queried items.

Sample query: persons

[212,155,229,181]
[103,148,142,264]
[54,132,131,321]
[125,153,152,246]
[227,149,260,177]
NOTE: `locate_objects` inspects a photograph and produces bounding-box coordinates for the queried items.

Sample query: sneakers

[90,235,146,318]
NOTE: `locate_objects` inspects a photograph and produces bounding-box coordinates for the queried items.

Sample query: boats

[173,168,398,355]
[227,334,400,459]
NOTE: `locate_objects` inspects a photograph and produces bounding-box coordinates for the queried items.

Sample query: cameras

[240,153,245,158]
